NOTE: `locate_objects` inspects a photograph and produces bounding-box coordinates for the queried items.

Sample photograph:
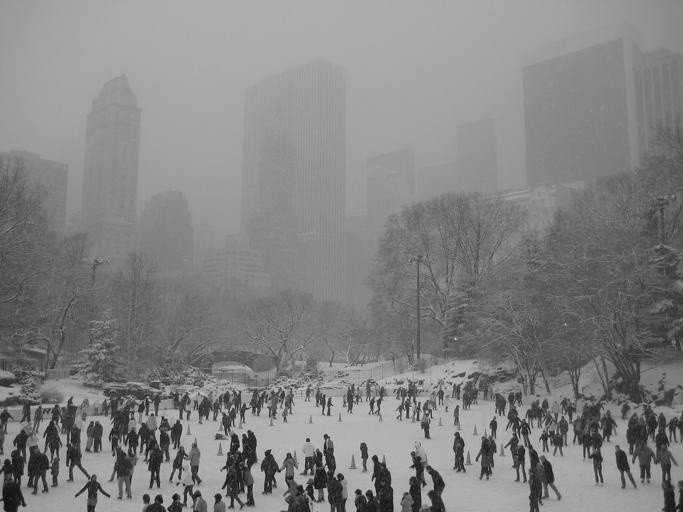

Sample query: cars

[69,359,87,376]
[175,383,199,397]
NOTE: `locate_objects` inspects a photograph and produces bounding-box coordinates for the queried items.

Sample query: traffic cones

[268,415,273,426]
[337,412,342,422]
[498,443,505,457]
[184,423,191,435]
[455,422,463,430]
[308,416,313,424]
[194,436,198,448]
[216,441,223,456]
[377,412,383,422]
[481,427,487,440]
[471,423,478,435]
[464,449,472,465]
[217,421,223,431]
[423,453,430,468]
[236,418,243,429]
[292,449,299,464]
[436,416,443,426]
[382,454,387,471]
[347,454,358,469]
[411,415,415,423]
[487,431,492,440]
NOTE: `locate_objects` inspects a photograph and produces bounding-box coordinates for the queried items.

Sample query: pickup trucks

[102,381,161,403]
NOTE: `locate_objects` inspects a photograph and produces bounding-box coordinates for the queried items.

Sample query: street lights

[407,254,432,371]
[641,191,676,354]
[82,257,110,360]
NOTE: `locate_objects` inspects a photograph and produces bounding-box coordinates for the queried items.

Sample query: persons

[0,381,682,511]
